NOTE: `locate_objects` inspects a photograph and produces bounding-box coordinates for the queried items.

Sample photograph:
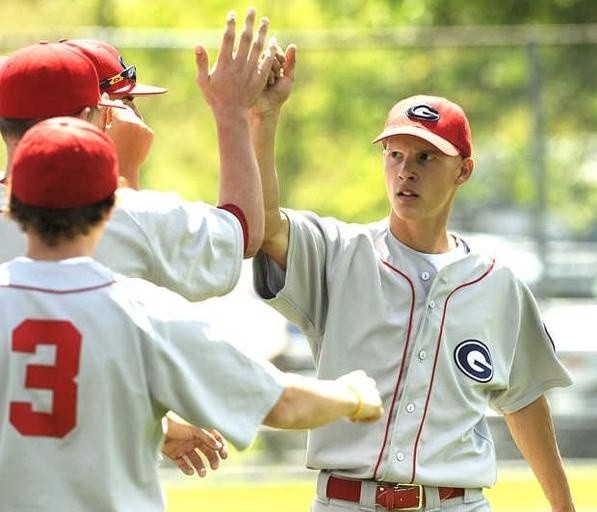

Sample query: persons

[48,37,231,478]
[229,42,577,512]
[1,114,387,512]
[0,6,278,307]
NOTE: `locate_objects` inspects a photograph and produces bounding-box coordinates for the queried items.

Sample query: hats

[371,94,473,160]
[0,40,126,120]
[0,116,117,210]
[58,39,168,96]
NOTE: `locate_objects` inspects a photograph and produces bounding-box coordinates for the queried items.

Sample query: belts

[325,477,465,512]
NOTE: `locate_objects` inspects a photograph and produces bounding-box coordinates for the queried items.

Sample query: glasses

[99,65,136,88]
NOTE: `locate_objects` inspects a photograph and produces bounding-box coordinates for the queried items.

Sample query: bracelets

[332,377,365,422]
